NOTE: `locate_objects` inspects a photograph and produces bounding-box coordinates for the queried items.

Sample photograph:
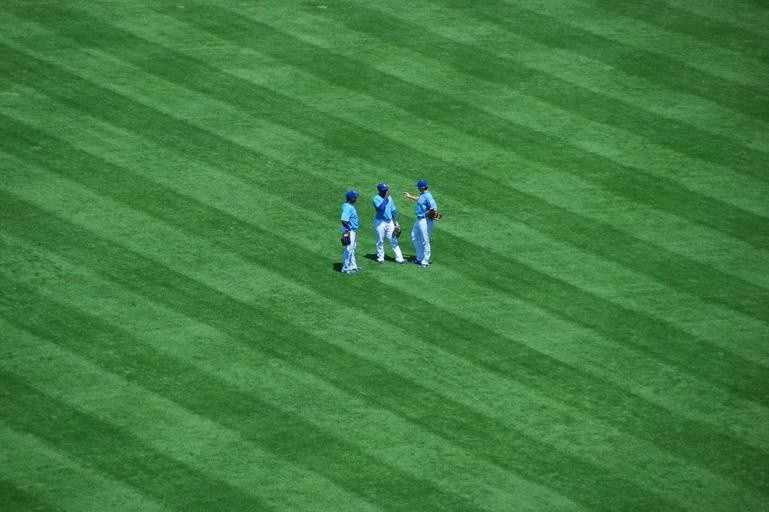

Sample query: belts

[418,216,423,219]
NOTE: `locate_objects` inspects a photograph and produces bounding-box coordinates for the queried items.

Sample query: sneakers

[342,267,361,273]
[399,261,407,264]
[411,260,428,268]
[380,261,384,264]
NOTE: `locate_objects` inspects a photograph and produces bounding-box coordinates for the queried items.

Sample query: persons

[339,190,362,273]
[372,183,408,264]
[403,179,442,268]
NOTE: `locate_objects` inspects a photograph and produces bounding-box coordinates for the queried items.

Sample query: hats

[378,183,389,191]
[416,180,429,186]
[347,191,359,199]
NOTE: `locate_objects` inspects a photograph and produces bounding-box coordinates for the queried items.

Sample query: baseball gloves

[392,226,401,238]
[341,231,351,246]
[428,208,440,221]
